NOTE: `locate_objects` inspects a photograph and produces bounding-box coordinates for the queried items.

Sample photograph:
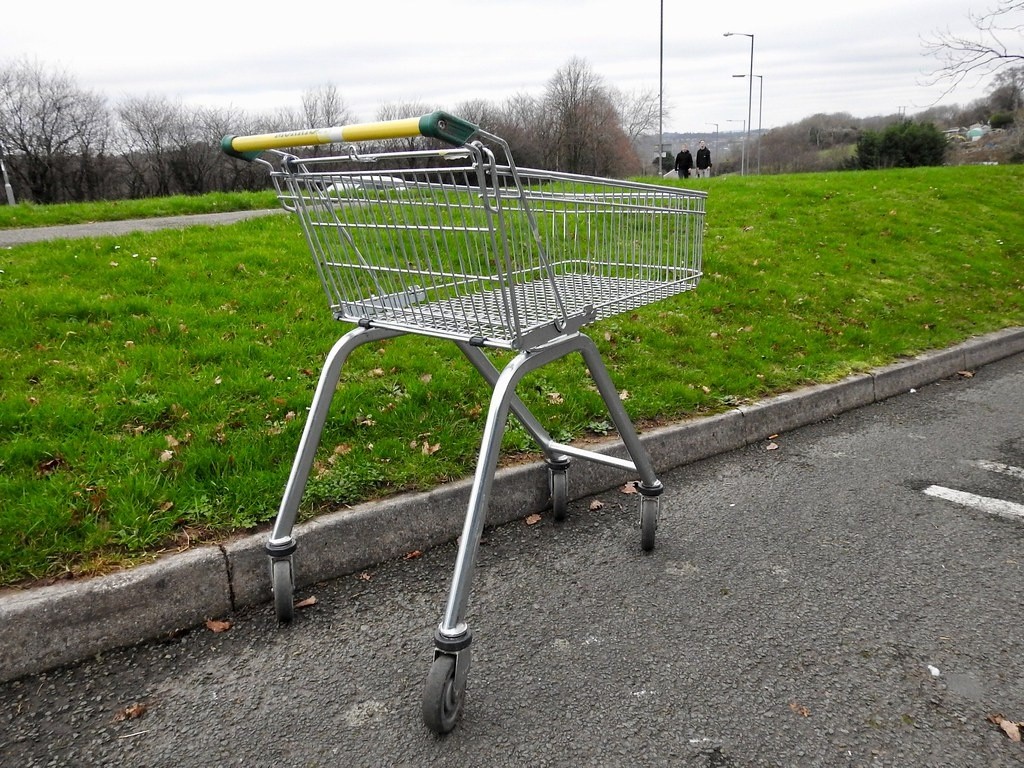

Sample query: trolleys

[220,110,708,734]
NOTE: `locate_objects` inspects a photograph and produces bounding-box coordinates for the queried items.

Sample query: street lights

[732,73,763,175]
[704,122,719,176]
[726,119,746,175]
[723,32,755,178]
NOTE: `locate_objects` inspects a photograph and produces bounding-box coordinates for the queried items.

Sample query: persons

[675,143,693,180]
[696,139,712,179]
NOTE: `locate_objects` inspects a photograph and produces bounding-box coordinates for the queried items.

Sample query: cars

[322,175,409,193]
